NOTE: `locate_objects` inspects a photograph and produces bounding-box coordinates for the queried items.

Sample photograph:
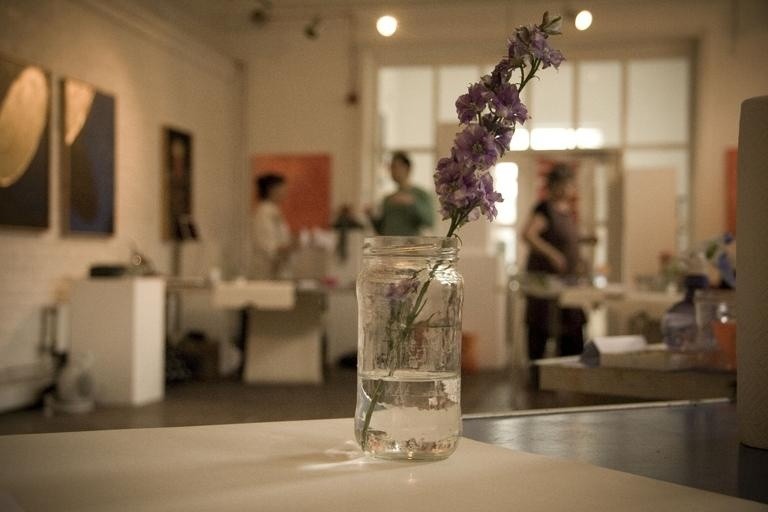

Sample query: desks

[1,399,737,510]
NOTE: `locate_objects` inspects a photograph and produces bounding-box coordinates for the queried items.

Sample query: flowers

[434,13,564,238]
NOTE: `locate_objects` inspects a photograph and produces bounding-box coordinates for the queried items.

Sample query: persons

[245,170,300,283]
[360,151,435,236]
[521,161,588,387]
